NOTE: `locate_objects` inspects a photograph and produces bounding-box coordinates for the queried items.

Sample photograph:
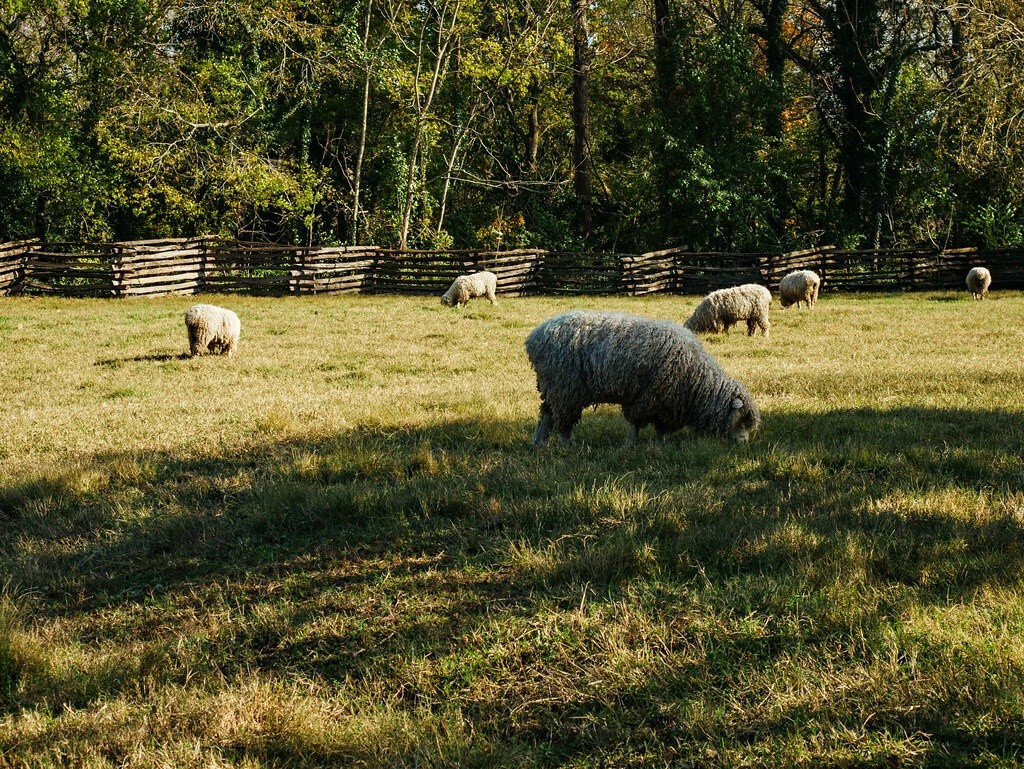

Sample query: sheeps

[524,309,760,448]
[184,303,241,359]
[966,267,991,301]
[683,283,773,338]
[778,269,821,311]
[440,271,497,310]
